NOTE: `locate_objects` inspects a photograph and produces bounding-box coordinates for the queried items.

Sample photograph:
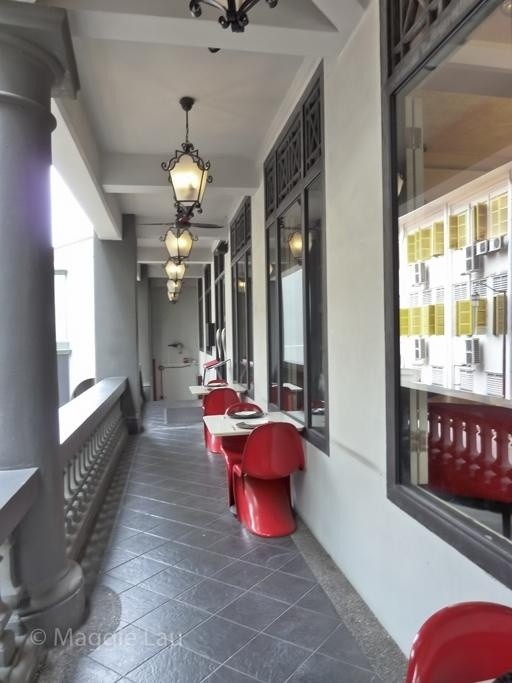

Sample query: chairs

[403,600,512,683]
[231,424,308,539]
[190,359,304,488]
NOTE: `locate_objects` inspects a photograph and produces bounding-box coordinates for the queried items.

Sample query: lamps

[288,224,312,266]
[160,98,215,216]
[157,215,199,305]
[189,0,278,32]
